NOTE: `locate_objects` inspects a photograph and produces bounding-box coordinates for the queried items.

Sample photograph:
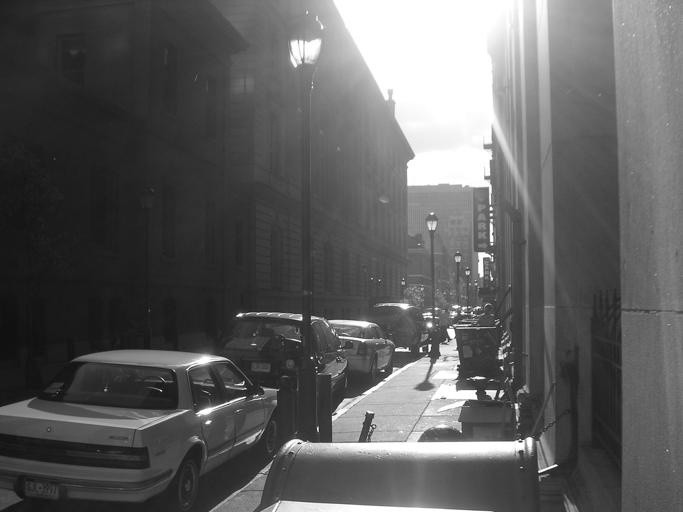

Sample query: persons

[476,304,494,328]
[437,308,454,346]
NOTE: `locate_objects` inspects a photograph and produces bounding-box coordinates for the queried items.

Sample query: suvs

[217,311,354,416]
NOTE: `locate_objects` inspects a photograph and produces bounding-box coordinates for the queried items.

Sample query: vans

[368,299,431,358]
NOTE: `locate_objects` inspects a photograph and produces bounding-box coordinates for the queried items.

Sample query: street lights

[136,181,155,349]
[400,276,405,303]
[285,8,333,443]
[368,273,374,305]
[425,212,442,357]
[464,265,470,307]
[453,250,460,305]
[474,280,478,305]
[377,274,383,304]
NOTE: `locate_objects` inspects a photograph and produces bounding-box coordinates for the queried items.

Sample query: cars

[327,317,397,381]
[421,304,482,330]
[0,348,281,511]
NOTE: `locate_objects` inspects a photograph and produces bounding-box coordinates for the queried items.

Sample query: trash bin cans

[452,324,499,371]
